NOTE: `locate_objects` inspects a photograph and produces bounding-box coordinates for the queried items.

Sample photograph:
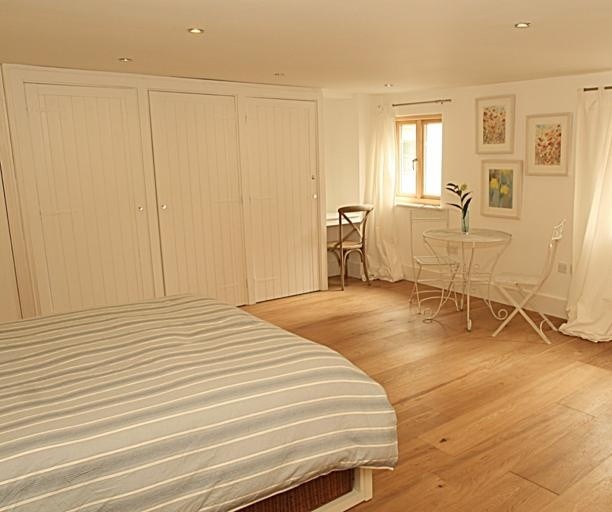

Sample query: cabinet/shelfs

[2,63,327,308]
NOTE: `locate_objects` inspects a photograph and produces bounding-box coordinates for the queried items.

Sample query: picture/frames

[474,92,572,220]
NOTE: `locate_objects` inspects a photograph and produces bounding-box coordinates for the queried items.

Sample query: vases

[460,210,469,235]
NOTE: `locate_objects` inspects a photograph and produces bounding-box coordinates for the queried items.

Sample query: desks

[421,228,512,333]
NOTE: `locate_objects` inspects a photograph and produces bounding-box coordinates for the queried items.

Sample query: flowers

[443,181,473,232]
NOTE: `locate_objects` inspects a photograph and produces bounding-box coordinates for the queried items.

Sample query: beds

[0,292,373,511]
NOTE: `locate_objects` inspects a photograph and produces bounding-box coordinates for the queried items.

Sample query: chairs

[408,207,460,314]
[489,218,566,346]
[326,205,374,291]
